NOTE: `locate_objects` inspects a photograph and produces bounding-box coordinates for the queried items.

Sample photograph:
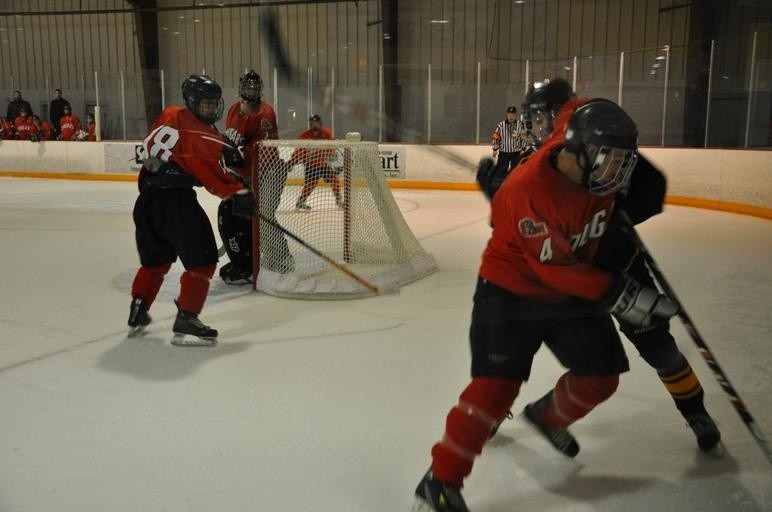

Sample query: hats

[506,106,516,112]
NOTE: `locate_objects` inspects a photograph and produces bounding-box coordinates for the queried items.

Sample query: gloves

[233,190,256,218]
[610,277,679,333]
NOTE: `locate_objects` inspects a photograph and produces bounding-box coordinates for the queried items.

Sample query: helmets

[310,113,320,121]
[566,98,639,196]
[181,75,224,122]
[522,78,572,147]
[239,69,262,101]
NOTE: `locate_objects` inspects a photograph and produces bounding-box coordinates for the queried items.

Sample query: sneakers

[685,411,720,451]
[415,468,468,511]
[221,262,251,280]
[296,203,311,209]
[524,392,581,458]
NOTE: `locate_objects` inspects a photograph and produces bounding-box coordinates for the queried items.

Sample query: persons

[411,100,677,509]
[223,70,296,283]
[0,89,96,142]
[491,106,519,171]
[477,79,723,452]
[127,74,256,348]
[284,116,346,210]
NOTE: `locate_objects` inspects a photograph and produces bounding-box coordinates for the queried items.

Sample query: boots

[172,299,216,336]
[129,296,152,325]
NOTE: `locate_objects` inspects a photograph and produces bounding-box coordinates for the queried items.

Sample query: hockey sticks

[259,7,478,174]
[258,212,399,295]
